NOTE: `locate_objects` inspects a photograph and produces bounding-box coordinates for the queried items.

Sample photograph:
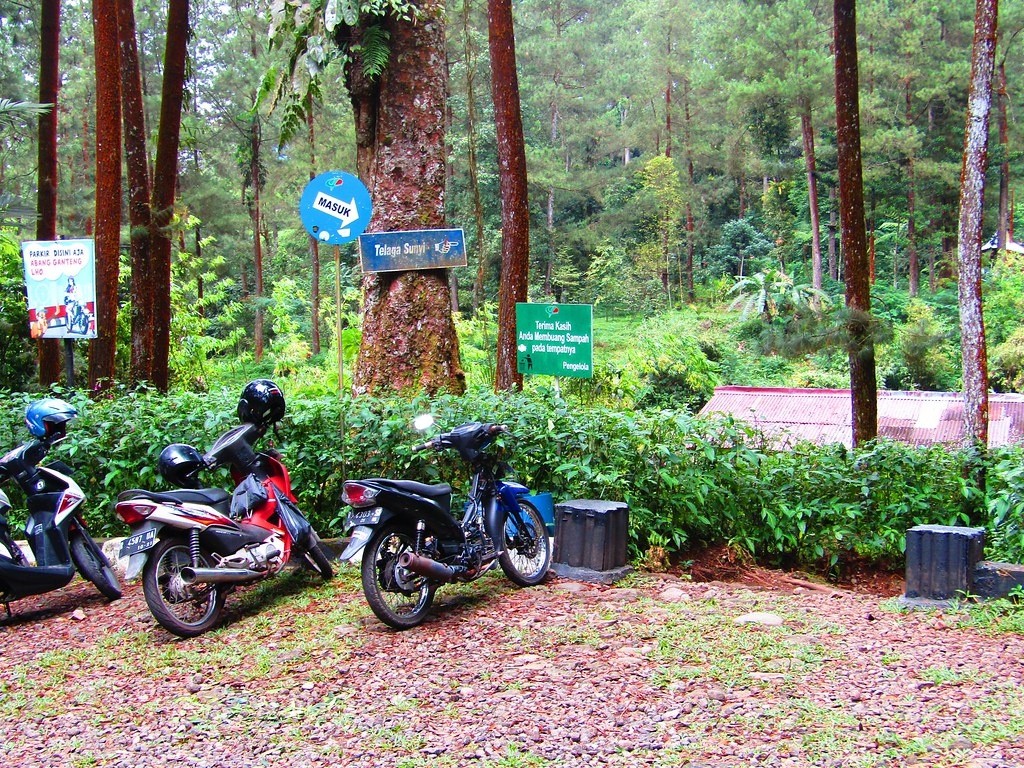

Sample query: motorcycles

[113,380,333,636]
[335,412,553,630]
[1,399,120,607]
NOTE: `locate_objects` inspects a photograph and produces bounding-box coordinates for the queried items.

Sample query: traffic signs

[297,170,376,246]
[358,226,469,278]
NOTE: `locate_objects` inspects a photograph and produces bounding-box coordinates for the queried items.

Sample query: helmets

[25,398,78,443]
[238,378,287,445]
[159,443,205,489]
